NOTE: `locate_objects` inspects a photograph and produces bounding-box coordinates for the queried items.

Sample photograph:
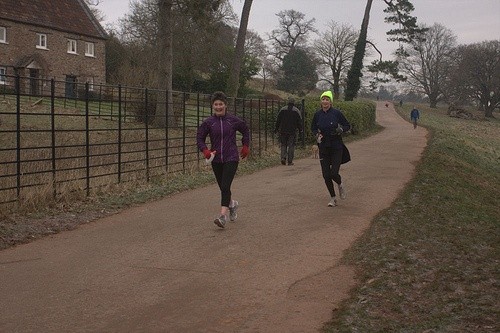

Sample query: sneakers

[338,183,345,199]
[229,200,239,221]
[328,199,336,207]
[214,214,226,228]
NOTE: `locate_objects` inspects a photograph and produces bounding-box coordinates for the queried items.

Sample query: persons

[310,90,352,207]
[197,91,250,228]
[400,98,403,107]
[411,107,420,128]
[275,96,304,166]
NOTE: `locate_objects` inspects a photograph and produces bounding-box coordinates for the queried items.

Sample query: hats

[211,91,226,103]
[320,91,332,102]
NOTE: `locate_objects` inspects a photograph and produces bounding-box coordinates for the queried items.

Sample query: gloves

[203,149,211,159]
[335,127,343,135]
[240,145,249,160]
